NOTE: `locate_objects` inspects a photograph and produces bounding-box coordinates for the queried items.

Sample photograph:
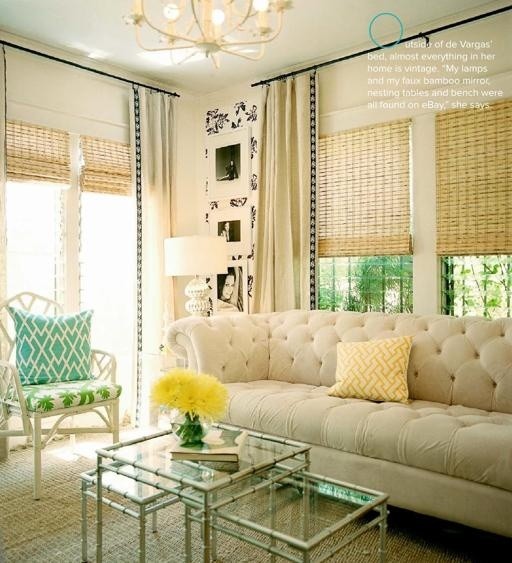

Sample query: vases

[169,407,216,442]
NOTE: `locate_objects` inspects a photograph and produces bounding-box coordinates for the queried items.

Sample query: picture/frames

[207,128,252,317]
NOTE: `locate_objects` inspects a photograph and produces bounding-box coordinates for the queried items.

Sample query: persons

[217,160,238,180]
[221,221,230,242]
[217,267,236,308]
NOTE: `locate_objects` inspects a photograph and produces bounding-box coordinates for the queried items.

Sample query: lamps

[121,0,294,67]
[163,234,228,317]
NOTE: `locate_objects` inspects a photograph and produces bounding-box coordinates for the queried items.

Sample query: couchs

[168,306,511,540]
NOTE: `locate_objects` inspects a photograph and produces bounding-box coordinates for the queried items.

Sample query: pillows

[331,334,415,402]
[6,303,96,385]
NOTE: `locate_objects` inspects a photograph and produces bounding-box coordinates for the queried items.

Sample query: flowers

[152,366,230,419]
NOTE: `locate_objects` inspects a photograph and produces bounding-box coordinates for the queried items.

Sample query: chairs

[0,291,121,500]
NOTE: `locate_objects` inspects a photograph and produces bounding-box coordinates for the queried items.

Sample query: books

[170,431,246,462]
